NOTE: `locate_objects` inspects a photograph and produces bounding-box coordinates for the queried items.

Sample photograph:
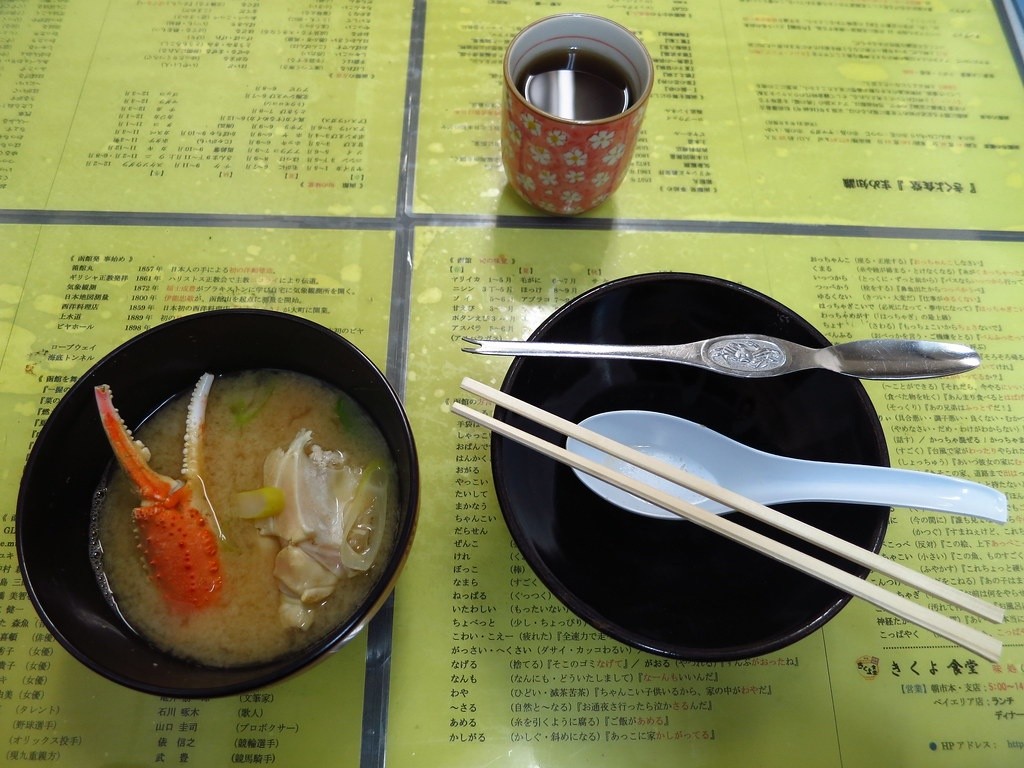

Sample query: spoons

[567,411,1008,523]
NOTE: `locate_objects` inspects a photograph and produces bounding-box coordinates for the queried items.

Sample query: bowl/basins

[15,310,419,698]
[490,273,890,661]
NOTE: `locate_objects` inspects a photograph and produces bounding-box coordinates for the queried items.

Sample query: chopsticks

[450,376,1004,665]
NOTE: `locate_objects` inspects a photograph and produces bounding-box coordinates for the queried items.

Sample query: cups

[504,14,655,214]
